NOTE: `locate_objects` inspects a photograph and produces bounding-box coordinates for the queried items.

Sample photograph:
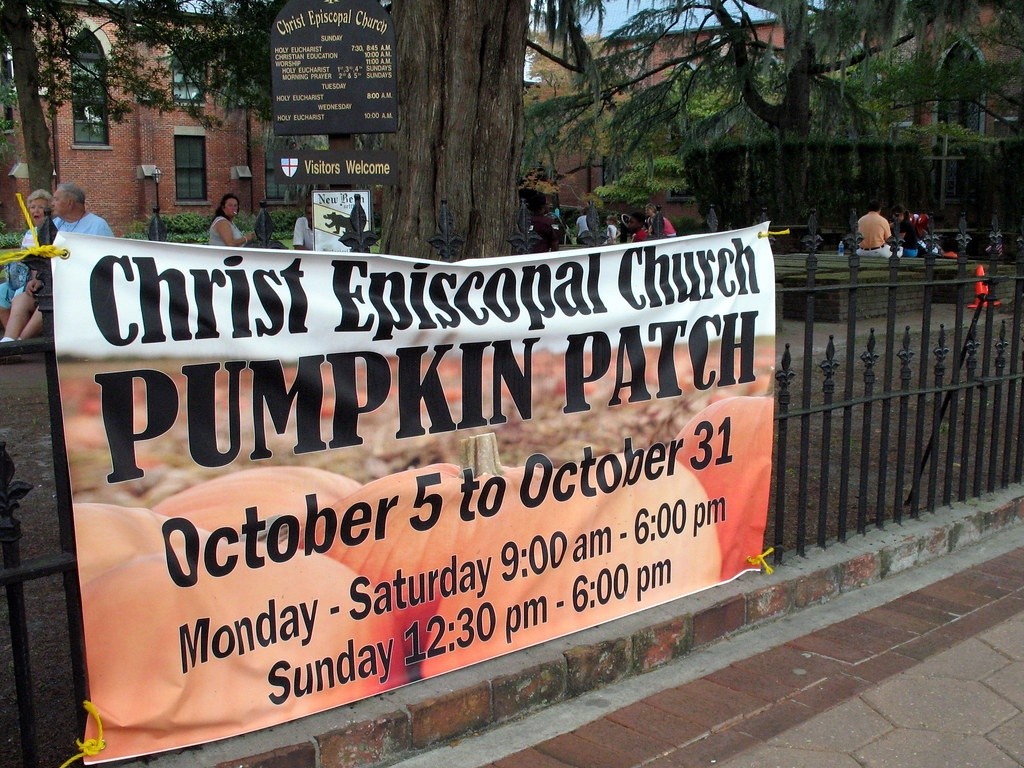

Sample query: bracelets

[243,235,247,242]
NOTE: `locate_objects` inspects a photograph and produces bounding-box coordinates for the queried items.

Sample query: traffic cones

[967,264,1001,308]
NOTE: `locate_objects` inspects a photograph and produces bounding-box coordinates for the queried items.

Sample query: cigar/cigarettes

[233,211,237,215]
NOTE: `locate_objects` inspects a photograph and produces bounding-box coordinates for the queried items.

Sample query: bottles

[839,241,844,256]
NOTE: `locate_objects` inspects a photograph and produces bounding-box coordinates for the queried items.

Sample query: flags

[985,238,1003,257]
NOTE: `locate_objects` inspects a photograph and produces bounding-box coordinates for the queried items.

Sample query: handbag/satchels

[5,262,29,302]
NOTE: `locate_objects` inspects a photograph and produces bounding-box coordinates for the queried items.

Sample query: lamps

[136,165,156,180]
[7,163,57,179]
[230,165,252,181]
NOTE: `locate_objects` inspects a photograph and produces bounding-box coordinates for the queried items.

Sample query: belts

[861,244,884,251]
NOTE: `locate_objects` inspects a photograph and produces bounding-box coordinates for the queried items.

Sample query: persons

[856,200,903,259]
[630,211,648,242]
[607,215,621,245]
[576,207,592,244]
[208,193,256,247]
[890,204,918,257]
[0,183,115,342]
[532,208,560,252]
[645,203,676,236]
[293,203,313,251]
